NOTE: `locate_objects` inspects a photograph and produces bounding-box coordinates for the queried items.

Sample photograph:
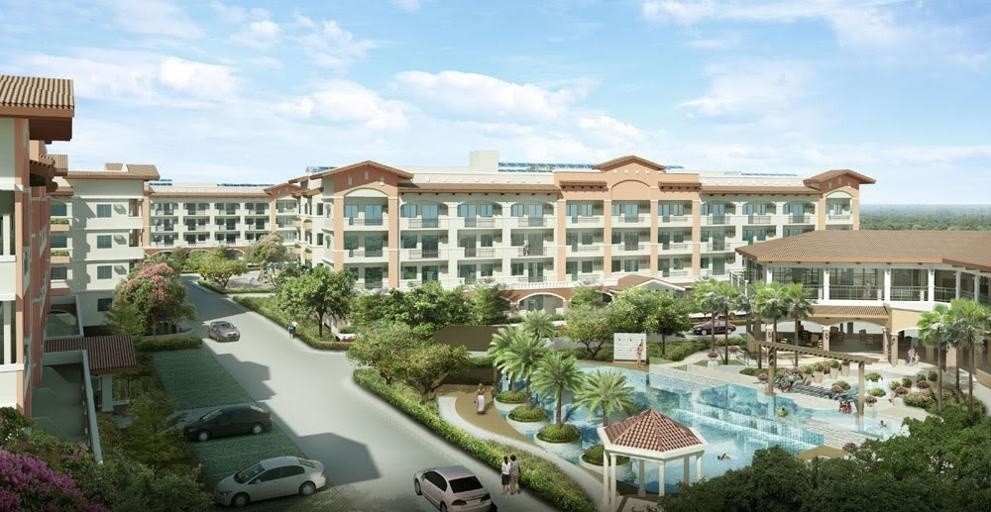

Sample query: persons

[837,400,853,414]
[777,368,804,393]
[631,344,644,369]
[509,454,521,496]
[907,345,920,367]
[526,241,531,256]
[878,420,887,430]
[288,320,294,340]
[500,455,511,495]
[522,241,527,256]
[776,407,785,417]
[474,383,486,415]
[717,452,732,461]
[291,319,298,338]
